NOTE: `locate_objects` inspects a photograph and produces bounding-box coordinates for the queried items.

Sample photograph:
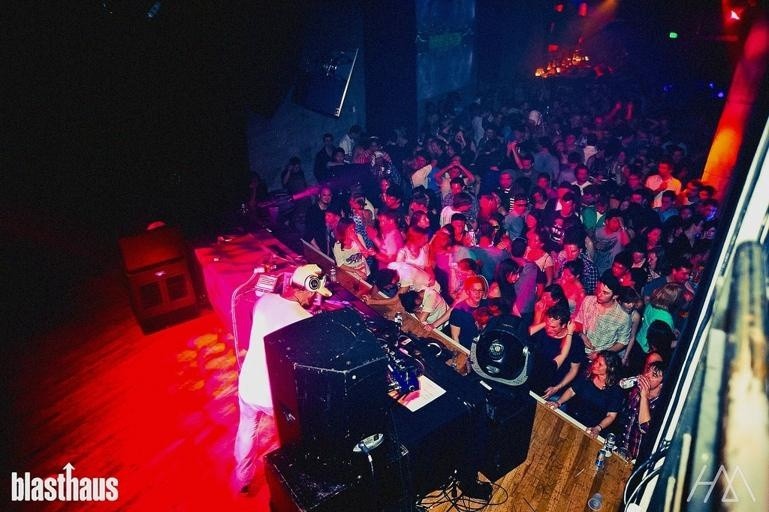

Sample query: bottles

[594,432,616,471]
[619,375,639,389]
[328,266,338,284]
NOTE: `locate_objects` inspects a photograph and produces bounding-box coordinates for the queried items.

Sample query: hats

[293,263,332,298]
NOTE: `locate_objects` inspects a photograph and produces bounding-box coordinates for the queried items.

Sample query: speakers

[262,305,536,511]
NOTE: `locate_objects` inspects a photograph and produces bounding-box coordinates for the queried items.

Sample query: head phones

[304,265,326,293]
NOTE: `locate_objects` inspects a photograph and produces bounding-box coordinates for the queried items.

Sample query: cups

[589,492,602,512]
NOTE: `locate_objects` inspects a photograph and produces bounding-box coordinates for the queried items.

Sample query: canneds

[595,449,606,467]
[394,312,403,326]
[603,432,616,458]
[469,231,476,246]
[619,375,642,390]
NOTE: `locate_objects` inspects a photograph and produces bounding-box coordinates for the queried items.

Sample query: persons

[233,65,720,493]
